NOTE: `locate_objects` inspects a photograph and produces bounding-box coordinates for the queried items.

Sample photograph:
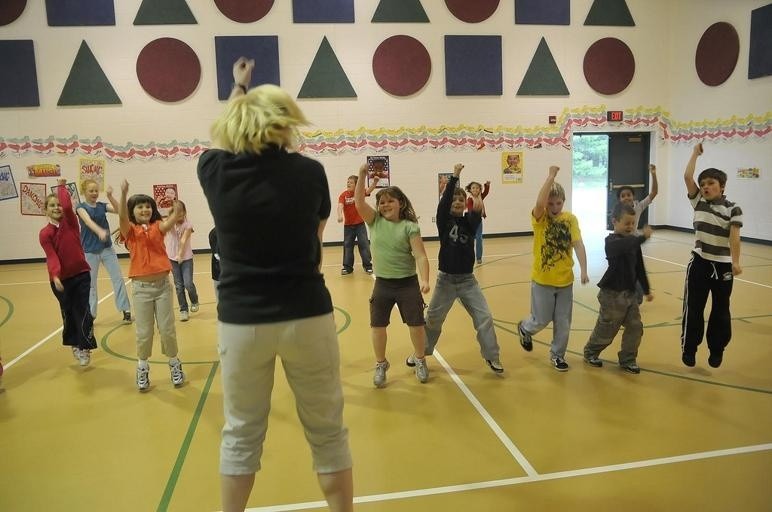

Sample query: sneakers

[476,257,483,264]
[68,343,92,367]
[580,351,603,367]
[340,265,373,276]
[122,310,132,324]
[707,342,724,368]
[617,355,640,374]
[681,341,696,367]
[414,354,429,384]
[169,357,186,387]
[405,349,421,366]
[482,356,504,374]
[177,302,200,321]
[517,321,533,352]
[550,353,570,372]
[372,358,392,387]
[135,362,151,390]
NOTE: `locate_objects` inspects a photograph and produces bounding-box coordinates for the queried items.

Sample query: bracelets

[230,81,249,96]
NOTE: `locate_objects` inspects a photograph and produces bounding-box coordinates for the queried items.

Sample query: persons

[516,141,657,375]
[679,141,744,371]
[196,56,356,511]
[37,178,200,391]
